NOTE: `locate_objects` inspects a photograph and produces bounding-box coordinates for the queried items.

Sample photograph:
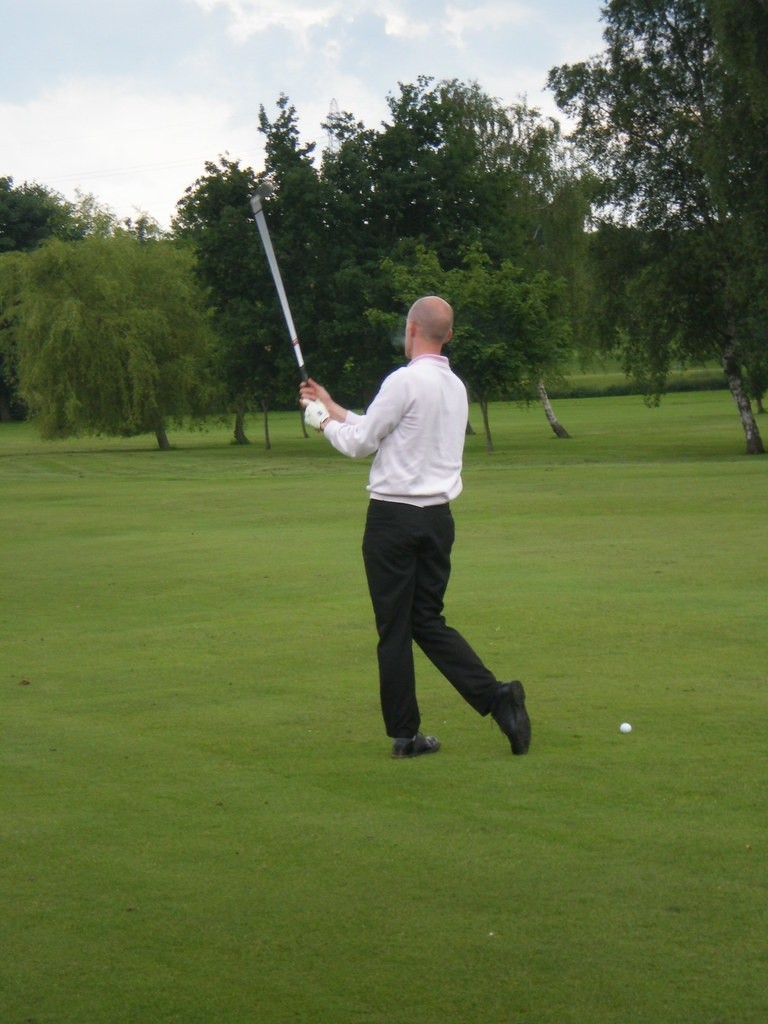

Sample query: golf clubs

[251,180,310,385]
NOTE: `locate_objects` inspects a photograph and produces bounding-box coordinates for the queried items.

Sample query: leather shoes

[490,680,531,753]
[391,731,440,758]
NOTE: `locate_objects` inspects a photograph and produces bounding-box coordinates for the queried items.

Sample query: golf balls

[619,722,633,735]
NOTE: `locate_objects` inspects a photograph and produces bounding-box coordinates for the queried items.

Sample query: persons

[299,298,536,757]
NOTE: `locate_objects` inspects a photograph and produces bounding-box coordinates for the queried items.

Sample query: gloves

[304,398,329,431]
